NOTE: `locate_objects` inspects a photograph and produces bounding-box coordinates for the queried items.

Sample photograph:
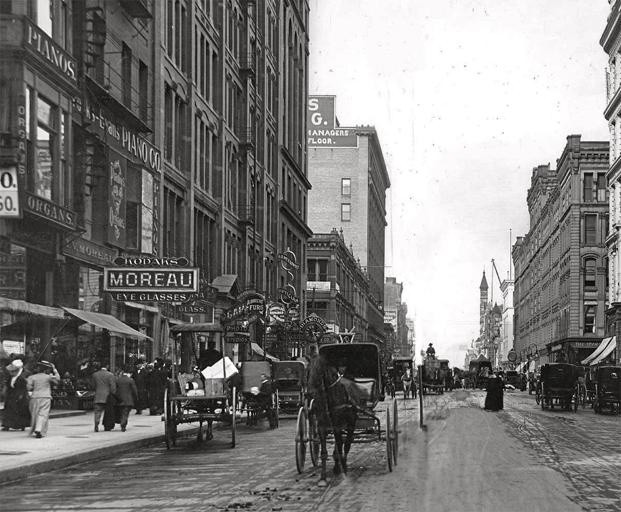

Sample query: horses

[303,352,361,487]
[402,367,412,399]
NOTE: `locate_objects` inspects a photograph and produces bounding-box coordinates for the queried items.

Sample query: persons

[0,351,173,437]
[336,360,355,380]
[198,339,223,381]
[427,343,435,359]
